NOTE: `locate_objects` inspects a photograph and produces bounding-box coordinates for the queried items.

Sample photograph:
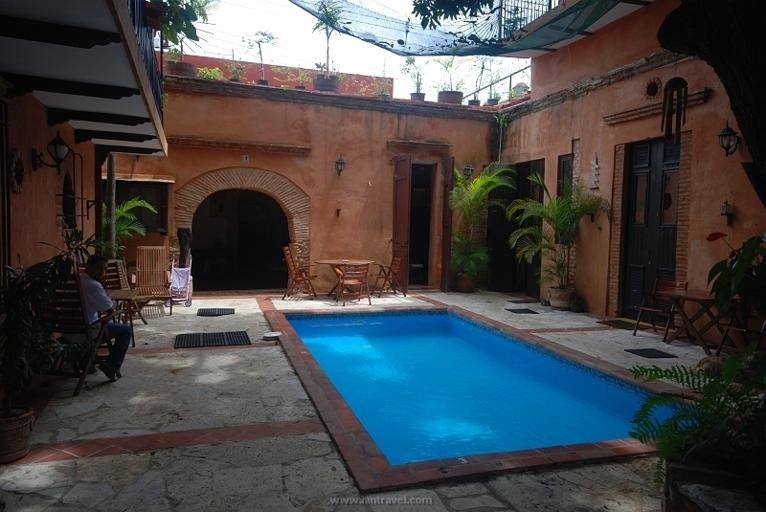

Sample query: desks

[659,290,738,355]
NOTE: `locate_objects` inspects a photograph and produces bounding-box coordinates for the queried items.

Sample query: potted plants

[310,1,351,92]
[455,161,513,293]
[269,57,313,90]
[240,28,280,86]
[0,264,48,464]
[164,1,216,78]
[469,55,531,107]
[376,63,391,98]
[432,56,466,105]
[223,58,245,82]
[398,53,430,101]
[509,175,609,310]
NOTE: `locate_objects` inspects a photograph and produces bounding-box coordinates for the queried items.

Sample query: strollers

[164,248,192,307]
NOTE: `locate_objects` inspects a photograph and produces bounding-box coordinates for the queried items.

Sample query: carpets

[172,331,251,349]
[596,317,650,329]
[197,308,234,316]
[506,299,539,303]
[626,349,677,359]
[507,306,538,316]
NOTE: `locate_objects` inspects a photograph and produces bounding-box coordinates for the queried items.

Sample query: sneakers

[99,362,121,382]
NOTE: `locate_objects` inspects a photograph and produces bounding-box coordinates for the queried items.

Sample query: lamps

[31,130,71,174]
[335,152,346,176]
[718,120,743,159]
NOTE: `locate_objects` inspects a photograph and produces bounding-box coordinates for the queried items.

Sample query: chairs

[280,239,407,306]
[41,245,176,395]
[718,301,766,352]
[632,277,689,342]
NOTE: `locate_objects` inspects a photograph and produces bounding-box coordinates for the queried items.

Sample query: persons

[59,254,131,382]
